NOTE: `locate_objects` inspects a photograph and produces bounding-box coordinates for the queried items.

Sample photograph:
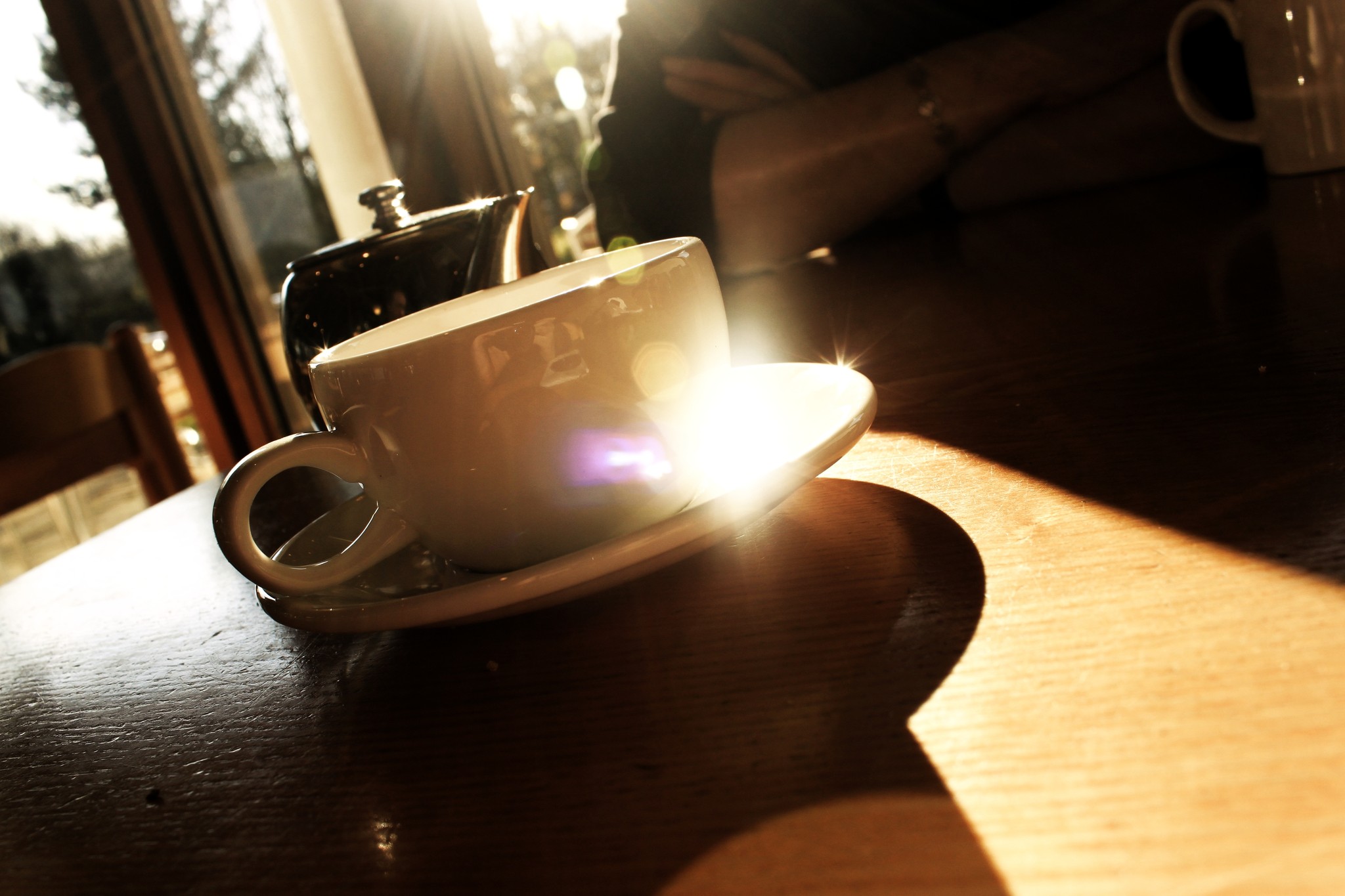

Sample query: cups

[1168,0,1345,176]
[209,233,739,595]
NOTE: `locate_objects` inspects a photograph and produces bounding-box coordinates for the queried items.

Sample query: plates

[256,364,880,632]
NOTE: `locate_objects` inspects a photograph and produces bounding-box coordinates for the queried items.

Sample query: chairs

[0,326,195,518]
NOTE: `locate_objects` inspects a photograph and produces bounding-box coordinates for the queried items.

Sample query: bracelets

[906,54,971,161]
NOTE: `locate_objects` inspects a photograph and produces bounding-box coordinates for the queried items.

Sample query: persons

[586,0,1256,256]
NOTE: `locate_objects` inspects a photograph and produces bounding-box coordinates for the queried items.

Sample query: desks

[0,169,1343,896]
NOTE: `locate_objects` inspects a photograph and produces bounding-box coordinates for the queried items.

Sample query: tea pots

[284,174,552,431]
